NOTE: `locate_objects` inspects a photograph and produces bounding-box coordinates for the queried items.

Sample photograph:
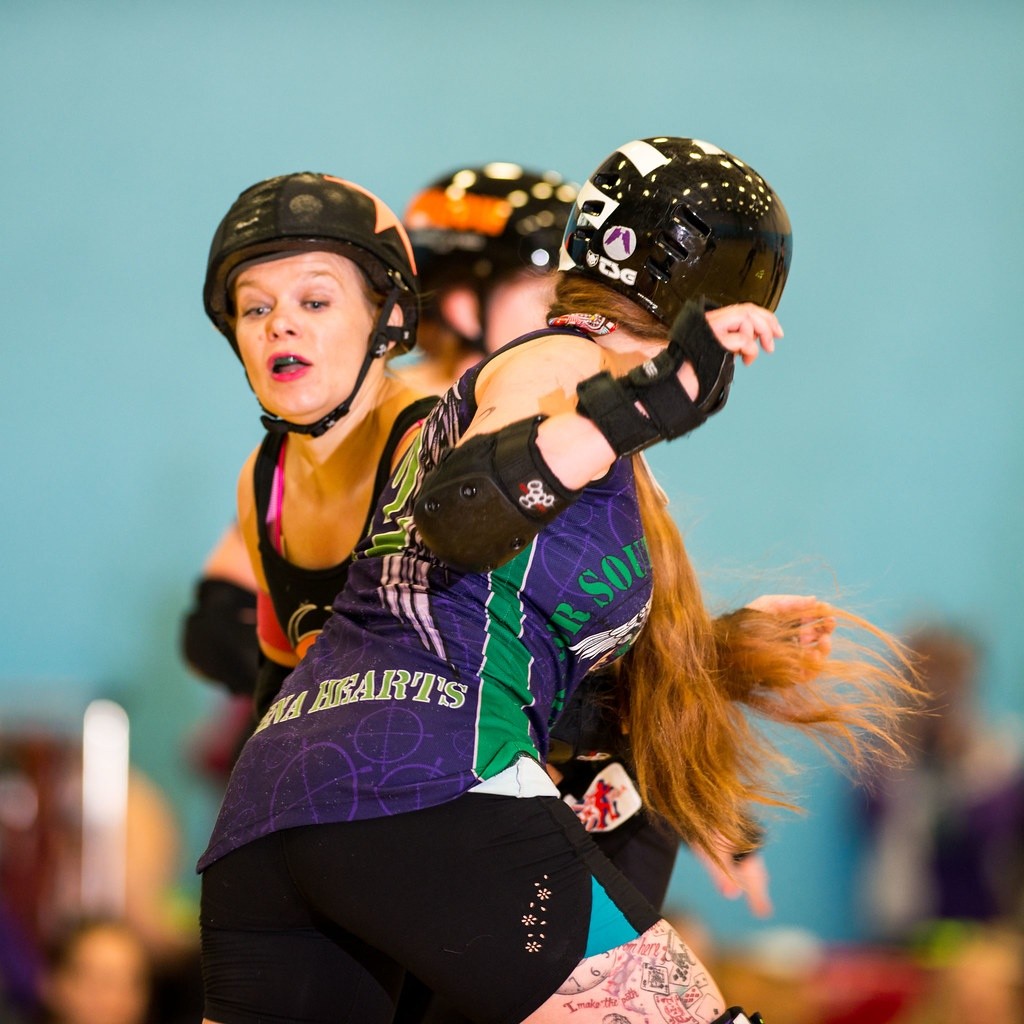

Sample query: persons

[1,732,200,1023]
[844,623,1024,946]
[176,160,770,919]
[196,136,835,1024]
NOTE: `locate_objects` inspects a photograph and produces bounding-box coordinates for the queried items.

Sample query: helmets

[402,161,582,265]
[204,173,419,358]
[559,138,792,360]
